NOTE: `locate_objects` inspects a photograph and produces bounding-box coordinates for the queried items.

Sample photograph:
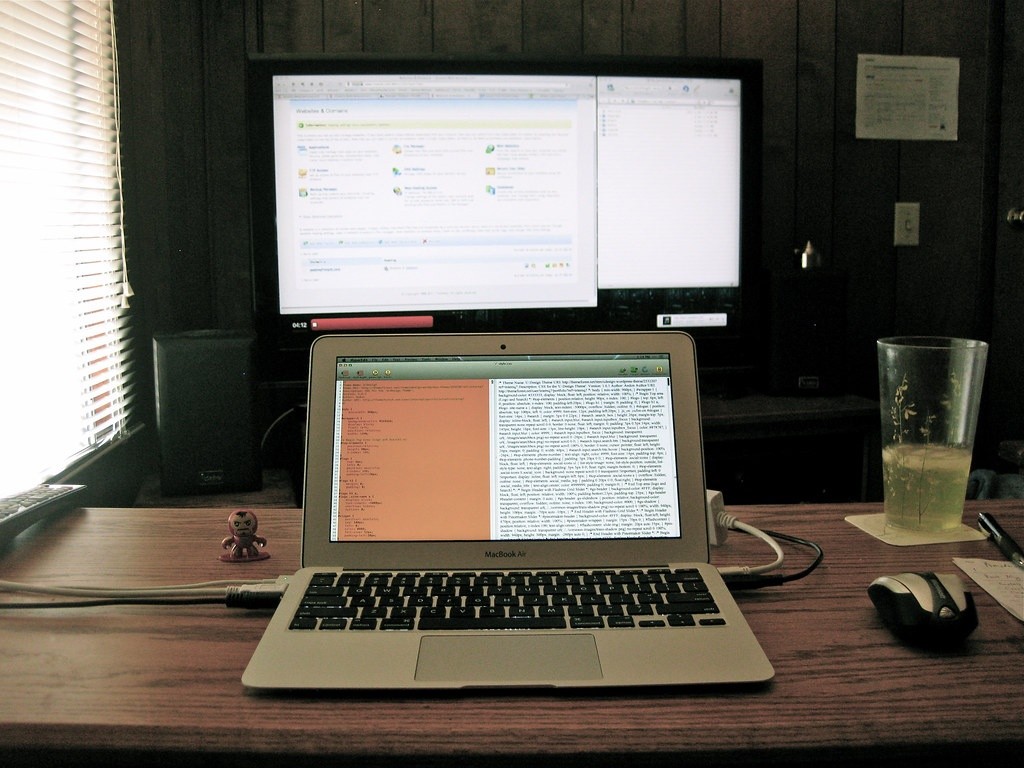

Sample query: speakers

[153,331,265,496]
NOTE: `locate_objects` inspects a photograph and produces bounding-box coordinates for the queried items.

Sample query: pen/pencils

[977,511,1024,569]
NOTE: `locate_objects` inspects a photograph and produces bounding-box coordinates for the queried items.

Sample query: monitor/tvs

[240,55,770,337]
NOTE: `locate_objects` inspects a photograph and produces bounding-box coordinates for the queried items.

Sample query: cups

[879,336,989,532]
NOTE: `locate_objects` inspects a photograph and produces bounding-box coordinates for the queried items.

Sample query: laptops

[241,335,776,692]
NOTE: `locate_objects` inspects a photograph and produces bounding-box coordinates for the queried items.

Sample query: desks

[0,499,1024,756]
[702,390,880,502]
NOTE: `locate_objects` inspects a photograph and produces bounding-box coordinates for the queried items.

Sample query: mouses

[869,572,978,650]
